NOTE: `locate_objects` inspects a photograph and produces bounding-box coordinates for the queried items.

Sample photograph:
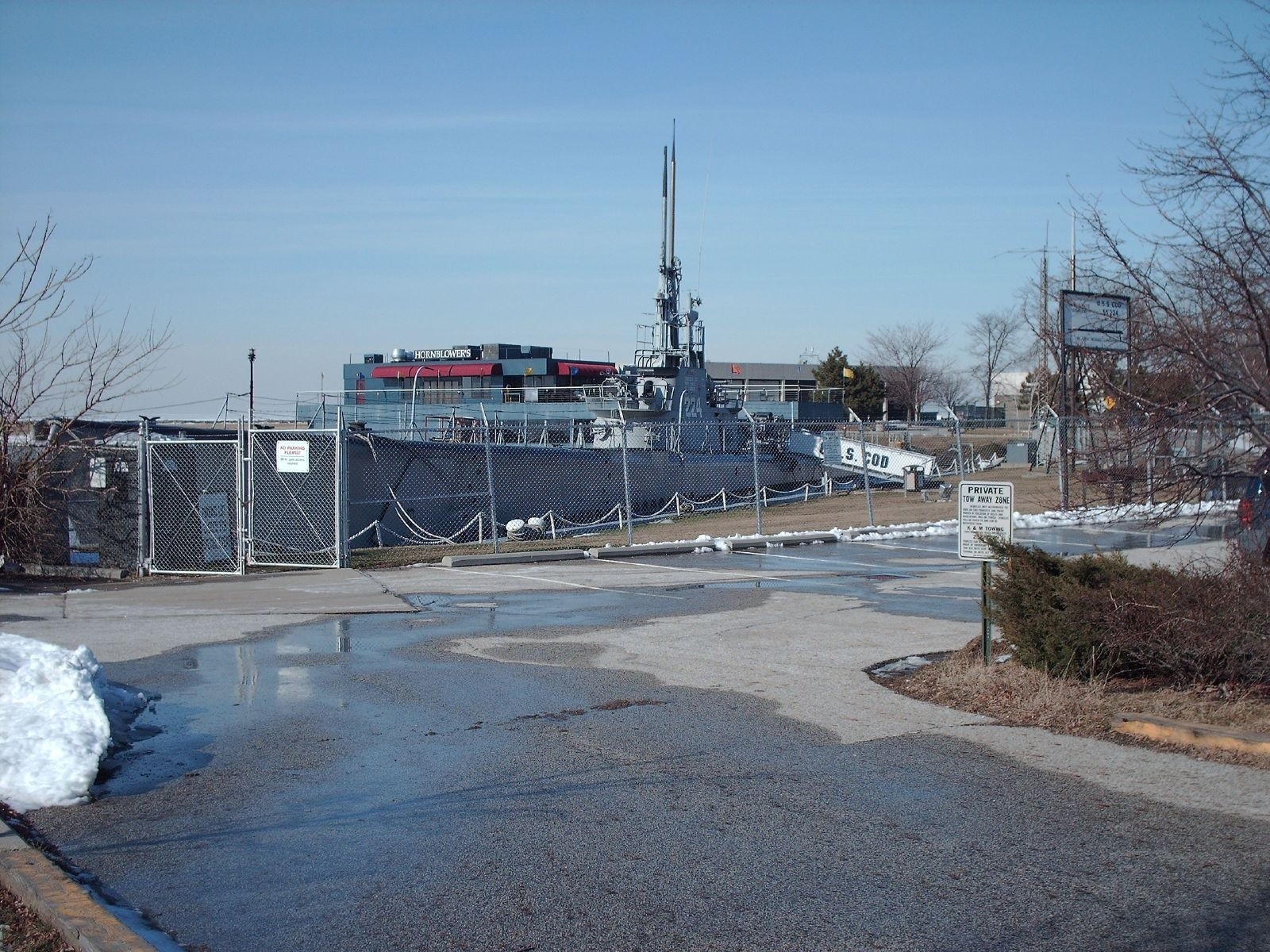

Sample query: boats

[33,116,784,549]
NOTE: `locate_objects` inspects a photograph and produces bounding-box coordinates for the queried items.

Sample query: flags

[572,367,578,375]
[844,368,854,378]
[526,368,533,374]
[732,363,743,374]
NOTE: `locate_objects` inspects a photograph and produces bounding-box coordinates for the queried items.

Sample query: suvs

[1236,443,1270,568]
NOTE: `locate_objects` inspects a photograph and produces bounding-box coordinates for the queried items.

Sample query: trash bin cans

[902,465,925,491]
